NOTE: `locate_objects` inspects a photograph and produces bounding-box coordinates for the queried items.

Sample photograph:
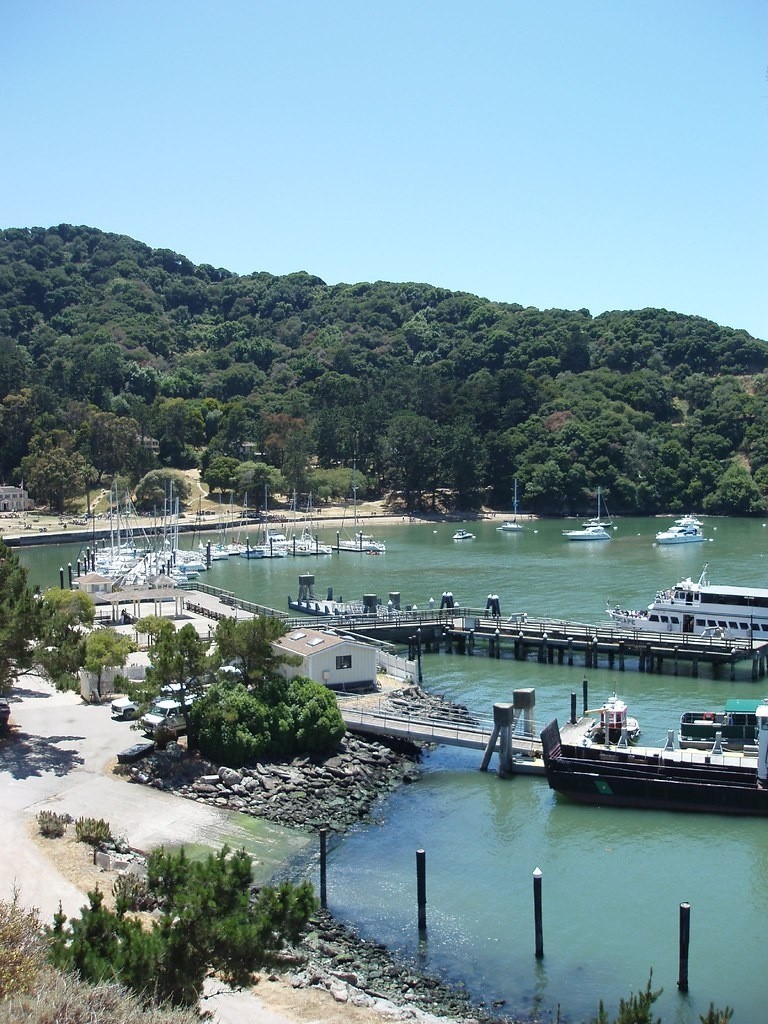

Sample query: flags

[19,481,22,490]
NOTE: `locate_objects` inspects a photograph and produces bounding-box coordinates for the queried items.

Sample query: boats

[495,523,523,530]
[654,515,707,544]
[584,691,641,746]
[451,528,473,539]
[676,698,768,751]
[539,718,768,815]
[560,524,612,540]
[603,564,768,640]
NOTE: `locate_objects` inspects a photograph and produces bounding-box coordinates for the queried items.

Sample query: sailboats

[502,478,517,523]
[337,430,386,553]
[581,484,614,528]
[68,476,334,587]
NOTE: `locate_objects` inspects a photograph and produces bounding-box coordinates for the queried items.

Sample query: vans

[139,697,194,735]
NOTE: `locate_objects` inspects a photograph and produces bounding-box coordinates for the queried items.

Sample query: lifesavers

[702,712,714,721]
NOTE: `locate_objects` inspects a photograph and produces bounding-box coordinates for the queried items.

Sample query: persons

[85,515,88,521]
[63,522,67,532]
[121,609,126,625]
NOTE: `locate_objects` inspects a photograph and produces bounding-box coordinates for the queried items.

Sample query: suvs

[110,682,184,721]
